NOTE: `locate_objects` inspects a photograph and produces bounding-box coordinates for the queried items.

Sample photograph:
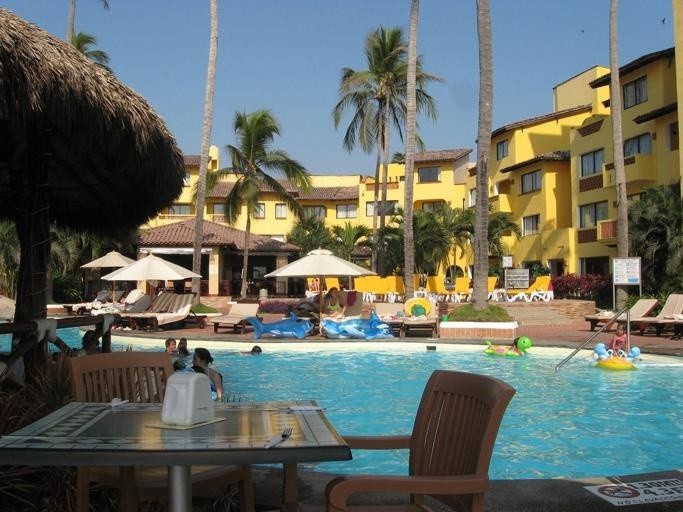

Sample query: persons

[325,287,346,319]
[241,345,262,355]
[608,323,627,357]
[509,337,519,351]
[162,336,223,402]
[309,278,319,291]
[77,331,100,357]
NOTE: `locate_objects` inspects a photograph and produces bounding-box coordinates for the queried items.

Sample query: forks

[263,423,294,450]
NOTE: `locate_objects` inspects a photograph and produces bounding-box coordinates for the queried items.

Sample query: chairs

[325,370,516,512]
[70,353,255,512]
[306,274,554,300]
[584,293,683,341]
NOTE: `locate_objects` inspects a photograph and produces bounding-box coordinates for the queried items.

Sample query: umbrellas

[100,251,202,313]
[80,249,137,303]
[264,248,378,336]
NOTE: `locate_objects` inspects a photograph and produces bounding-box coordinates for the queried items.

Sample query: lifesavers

[484,337,532,357]
[592,343,640,370]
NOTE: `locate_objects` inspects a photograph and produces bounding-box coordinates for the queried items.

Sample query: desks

[0,401,353,511]
